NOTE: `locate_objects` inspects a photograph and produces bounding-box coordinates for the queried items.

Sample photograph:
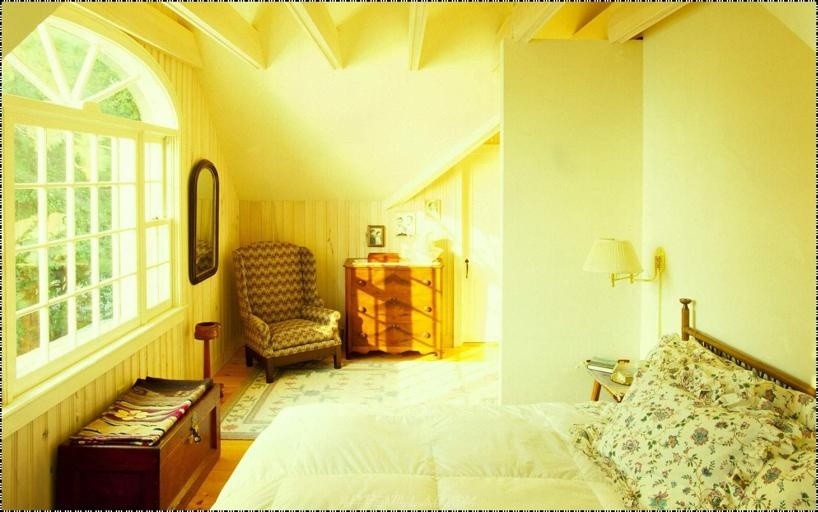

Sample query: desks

[583,360,642,401]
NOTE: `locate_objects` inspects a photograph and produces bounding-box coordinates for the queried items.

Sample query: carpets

[220,361,433,440]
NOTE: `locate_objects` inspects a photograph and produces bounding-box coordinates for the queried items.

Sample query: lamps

[582,235,665,288]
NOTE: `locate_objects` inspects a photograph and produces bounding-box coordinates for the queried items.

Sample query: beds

[209,298,816,510]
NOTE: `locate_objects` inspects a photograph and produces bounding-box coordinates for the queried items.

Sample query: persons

[396,215,414,236]
[370,231,381,245]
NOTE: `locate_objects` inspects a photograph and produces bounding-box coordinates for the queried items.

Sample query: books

[587,357,618,374]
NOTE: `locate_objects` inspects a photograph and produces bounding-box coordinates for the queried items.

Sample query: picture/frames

[368,225,384,247]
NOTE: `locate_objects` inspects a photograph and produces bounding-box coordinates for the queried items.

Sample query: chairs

[233,241,342,383]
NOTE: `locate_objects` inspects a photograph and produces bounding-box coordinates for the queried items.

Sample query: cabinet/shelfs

[58,384,221,510]
[343,258,449,360]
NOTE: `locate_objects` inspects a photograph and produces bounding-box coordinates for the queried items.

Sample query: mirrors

[188,159,219,285]
